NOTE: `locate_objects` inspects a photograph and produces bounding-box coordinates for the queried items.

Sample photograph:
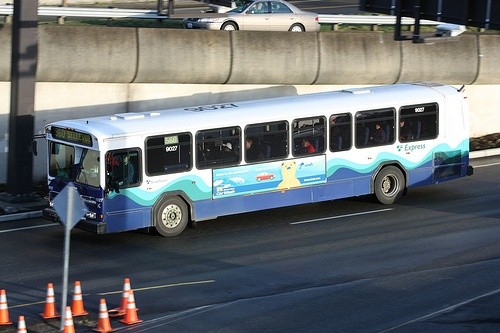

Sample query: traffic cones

[91,298,115,333]
[119,289,143,326]
[64,306,75,333]
[16,315,28,333]
[115,278,139,313]
[39,283,61,318]
[69,281,89,316]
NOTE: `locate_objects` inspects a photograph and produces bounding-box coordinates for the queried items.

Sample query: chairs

[260,120,422,160]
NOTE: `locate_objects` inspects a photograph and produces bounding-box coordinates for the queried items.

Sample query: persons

[302,137,316,154]
[245,138,265,163]
[251,2,265,14]
[369,122,387,144]
[117,154,134,186]
[399,120,409,141]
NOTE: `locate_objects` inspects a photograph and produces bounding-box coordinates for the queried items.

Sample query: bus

[40,80,474,236]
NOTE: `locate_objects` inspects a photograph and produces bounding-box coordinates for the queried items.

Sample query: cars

[185,1,320,35]
